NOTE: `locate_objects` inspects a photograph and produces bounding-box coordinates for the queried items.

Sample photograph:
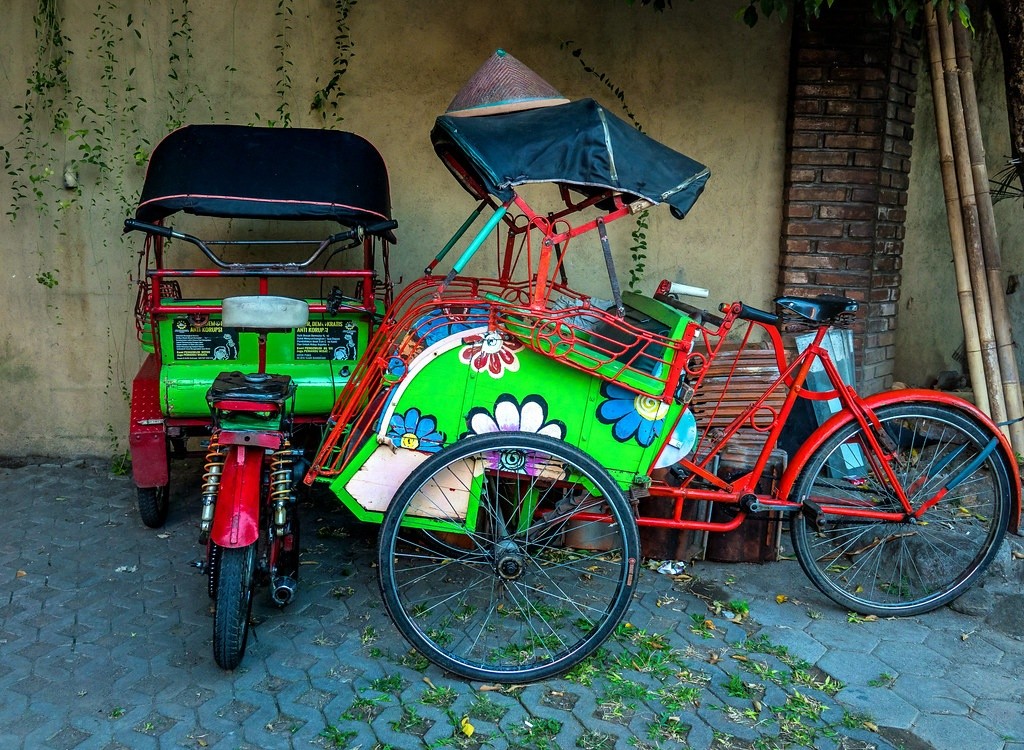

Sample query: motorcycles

[122,125,399,674]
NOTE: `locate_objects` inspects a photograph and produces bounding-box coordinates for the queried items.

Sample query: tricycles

[301,97,1021,684]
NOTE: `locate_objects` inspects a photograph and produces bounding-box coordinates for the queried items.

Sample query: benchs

[315,289,696,533]
[141,297,389,418]
